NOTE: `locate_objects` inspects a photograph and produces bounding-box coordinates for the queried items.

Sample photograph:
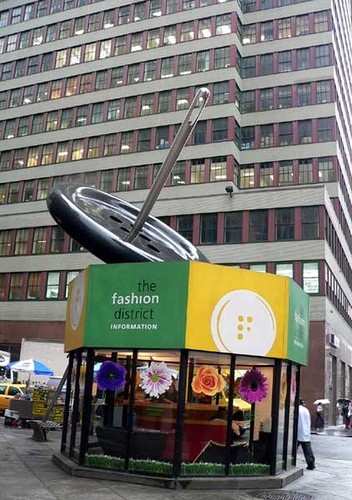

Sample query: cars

[0,383,32,411]
[211,382,251,433]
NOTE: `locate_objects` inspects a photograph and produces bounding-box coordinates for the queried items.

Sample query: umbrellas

[338,398,351,404]
[4,358,53,383]
[313,399,331,405]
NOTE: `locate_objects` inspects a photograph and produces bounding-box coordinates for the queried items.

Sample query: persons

[316,403,324,432]
[347,402,352,428]
[297,398,316,470]
[341,401,349,428]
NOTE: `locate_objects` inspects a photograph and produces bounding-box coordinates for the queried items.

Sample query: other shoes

[306,462,315,470]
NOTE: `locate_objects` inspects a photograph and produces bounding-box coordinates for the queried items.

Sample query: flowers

[95,360,128,391]
[239,367,268,404]
[139,361,173,399]
[191,366,227,397]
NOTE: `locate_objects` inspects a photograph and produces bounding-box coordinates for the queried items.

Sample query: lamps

[225,186,234,198]
